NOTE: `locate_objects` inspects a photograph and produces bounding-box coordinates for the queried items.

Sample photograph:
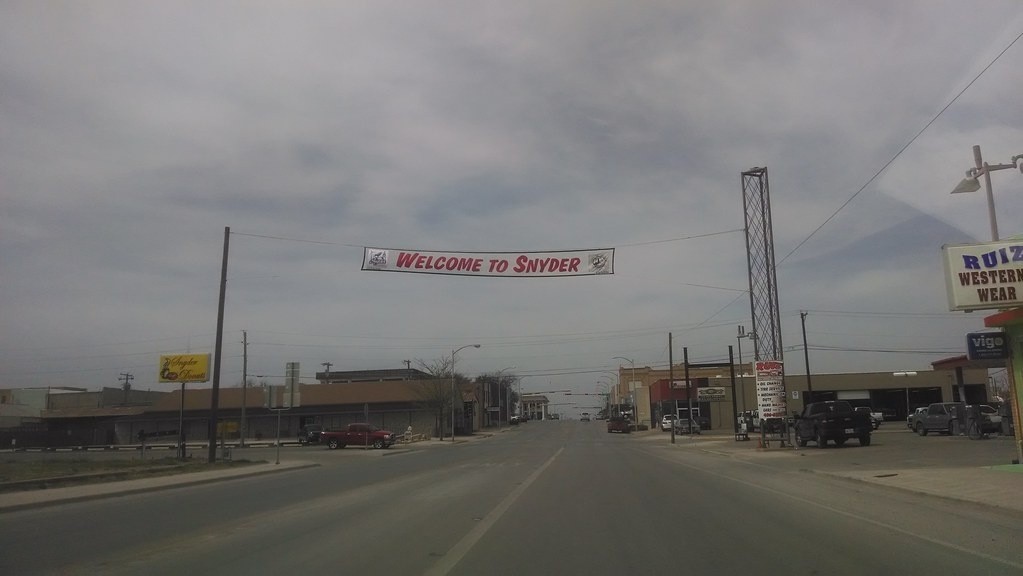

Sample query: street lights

[612,357,638,431]
[499,366,516,430]
[603,371,620,413]
[518,375,533,424]
[452,344,481,442]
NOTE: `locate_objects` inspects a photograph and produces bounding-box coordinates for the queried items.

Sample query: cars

[736,409,761,430]
[510,410,530,425]
[595,403,635,426]
[907,394,1005,437]
[606,416,630,434]
[763,417,786,434]
[297,423,327,444]
[551,414,559,420]
[579,412,591,421]
[660,414,680,432]
[693,416,711,430]
[674,418,701,436]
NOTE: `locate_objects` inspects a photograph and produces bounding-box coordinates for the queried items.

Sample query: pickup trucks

[851,406,884,430]
[793,400,874,449]
[320,422,396,450]
